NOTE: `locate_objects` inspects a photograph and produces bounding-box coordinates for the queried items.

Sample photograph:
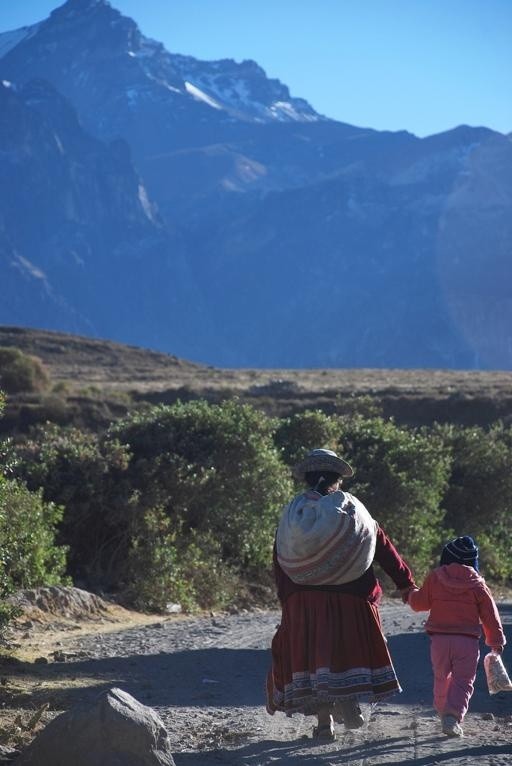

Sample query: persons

[404,536,507,736]
[264,448,420,743]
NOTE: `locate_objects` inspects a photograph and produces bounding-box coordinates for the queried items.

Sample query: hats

[297,450,353,477]
[440,534,480,572]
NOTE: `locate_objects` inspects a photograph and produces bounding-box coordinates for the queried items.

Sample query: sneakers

[442,718,463,736]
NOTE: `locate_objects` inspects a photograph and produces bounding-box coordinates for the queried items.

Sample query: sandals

[313,726,335,741]
[340,699,364,728]
